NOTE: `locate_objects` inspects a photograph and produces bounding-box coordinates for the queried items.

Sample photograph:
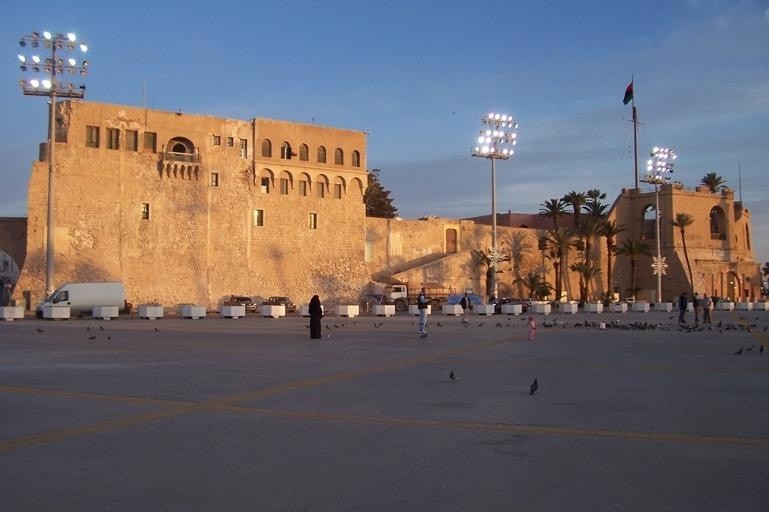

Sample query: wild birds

[541,319,769,334]
[425,318,527,333]
[85,326,113,341]
[735,341,766,357]
[304,318,384,333]
[36,328,44,333]
[449,369,456,381]
[529,378,539,395]
[411,319,417,326]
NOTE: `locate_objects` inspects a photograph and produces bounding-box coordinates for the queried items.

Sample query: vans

[477,298,532,315]
[35,281,126,318]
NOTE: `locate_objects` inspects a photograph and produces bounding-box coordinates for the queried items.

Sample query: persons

[460,292,472,323]
[677,291,688,323]
[702,294,714,323]
[307,295,323,339]
[693,291,704,325]
[418,287,433,338]
[527,316,537,341]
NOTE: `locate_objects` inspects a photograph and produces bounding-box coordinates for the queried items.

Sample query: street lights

[640,144,677,301]
[17,30,89,303]
[473,113,518,298]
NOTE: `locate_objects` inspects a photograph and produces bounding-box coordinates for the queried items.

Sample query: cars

[710,296,730,306]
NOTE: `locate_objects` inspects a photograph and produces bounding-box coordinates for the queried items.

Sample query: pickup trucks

[224,296,256,314]
[260,296,295,314]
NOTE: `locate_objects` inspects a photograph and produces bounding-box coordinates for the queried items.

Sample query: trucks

[382,284,451,311]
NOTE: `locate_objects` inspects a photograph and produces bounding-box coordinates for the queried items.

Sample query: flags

[622,80,632,105]
